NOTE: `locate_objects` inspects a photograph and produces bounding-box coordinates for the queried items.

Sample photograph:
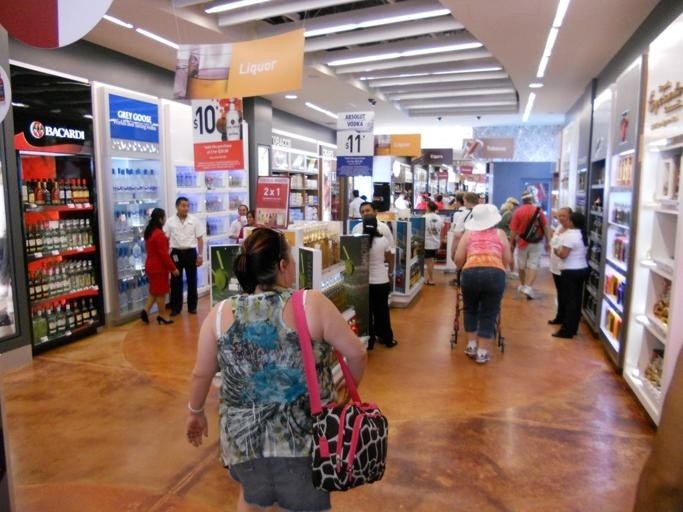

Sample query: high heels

[139,309,174,325]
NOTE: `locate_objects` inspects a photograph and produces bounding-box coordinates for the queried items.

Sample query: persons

[228,204,256,245]
[182,226,368,511]
[630,344,683,511]
[498,190,554,299]
[162,196,207,317]
[454,203,512,365]
[139,207,182,326]
[547,207,591,340]
[397,190,512,286]
[344,189,399,348]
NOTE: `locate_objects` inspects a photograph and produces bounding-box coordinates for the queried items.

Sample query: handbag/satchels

[309,401,388,491]
[519,208,544,243]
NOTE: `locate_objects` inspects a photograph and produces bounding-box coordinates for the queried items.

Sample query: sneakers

[517,284,535,299]
[387,340,397,347]
[464,344,490,363]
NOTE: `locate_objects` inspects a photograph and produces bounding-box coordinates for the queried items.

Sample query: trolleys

[449,266,505,354]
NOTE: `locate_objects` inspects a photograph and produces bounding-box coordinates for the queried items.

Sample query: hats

[521,191,533,200]
[463,204,503,232]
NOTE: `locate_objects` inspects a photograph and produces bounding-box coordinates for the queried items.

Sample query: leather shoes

[548,319,577,337]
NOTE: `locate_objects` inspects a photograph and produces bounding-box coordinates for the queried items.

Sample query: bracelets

[197,254,203,258]
[185,400,206,415]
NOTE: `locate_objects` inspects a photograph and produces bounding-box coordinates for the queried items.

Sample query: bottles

[112,168,158,303]
[320,274,351,315]
[585,187,601,316]
[302,232,341,266]
[604,156,630,342]
[205,173,224,236]
[21,177,97,342]
[174,168,199,213]
